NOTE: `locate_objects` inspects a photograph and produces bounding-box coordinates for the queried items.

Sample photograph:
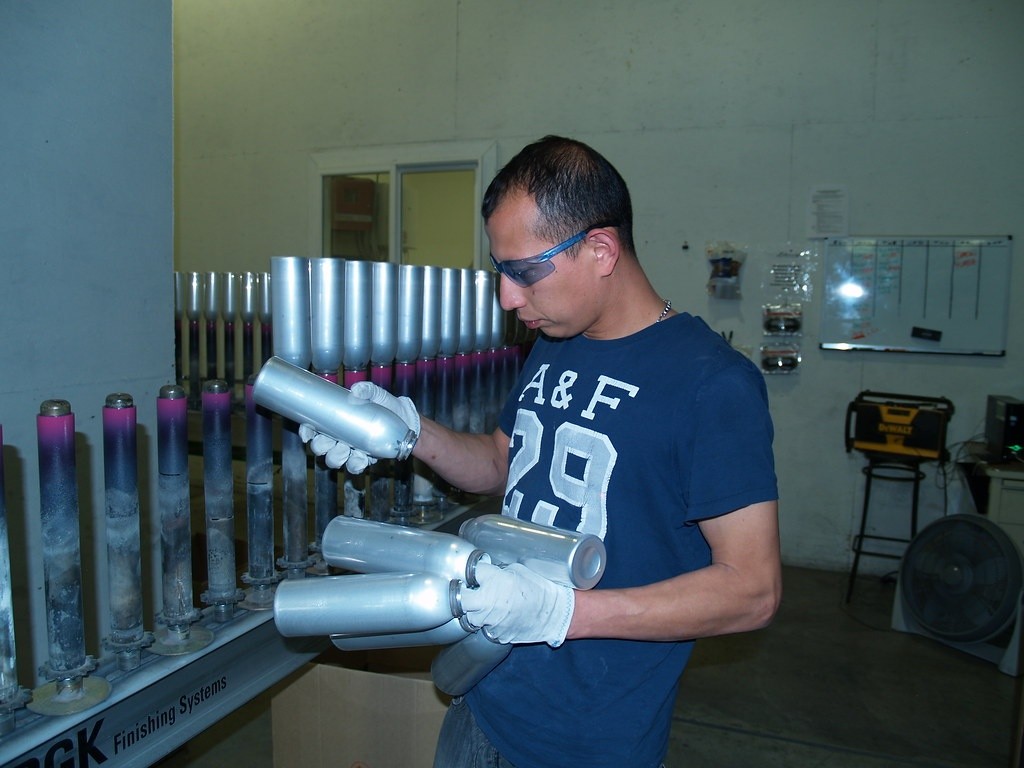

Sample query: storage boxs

[271,645,456,768]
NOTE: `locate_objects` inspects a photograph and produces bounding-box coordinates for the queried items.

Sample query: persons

[301,134,783,768]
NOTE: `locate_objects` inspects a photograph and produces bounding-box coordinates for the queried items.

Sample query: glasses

[488,221,622,288]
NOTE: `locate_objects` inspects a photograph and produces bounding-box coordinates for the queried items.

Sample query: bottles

[271,512,606,696]
[251,256,509,461]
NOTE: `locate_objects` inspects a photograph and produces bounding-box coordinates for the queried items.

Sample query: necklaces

[654,298,672,323]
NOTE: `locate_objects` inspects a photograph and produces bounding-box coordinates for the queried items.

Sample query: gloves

[461,562,575,648]
[298,381,421,475]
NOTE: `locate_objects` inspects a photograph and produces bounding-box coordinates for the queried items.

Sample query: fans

[891,513,1021,678]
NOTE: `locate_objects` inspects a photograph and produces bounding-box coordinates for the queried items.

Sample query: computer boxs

[984,394,1024,464]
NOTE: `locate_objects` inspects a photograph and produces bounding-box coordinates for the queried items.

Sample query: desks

[959,441,1023,559]
